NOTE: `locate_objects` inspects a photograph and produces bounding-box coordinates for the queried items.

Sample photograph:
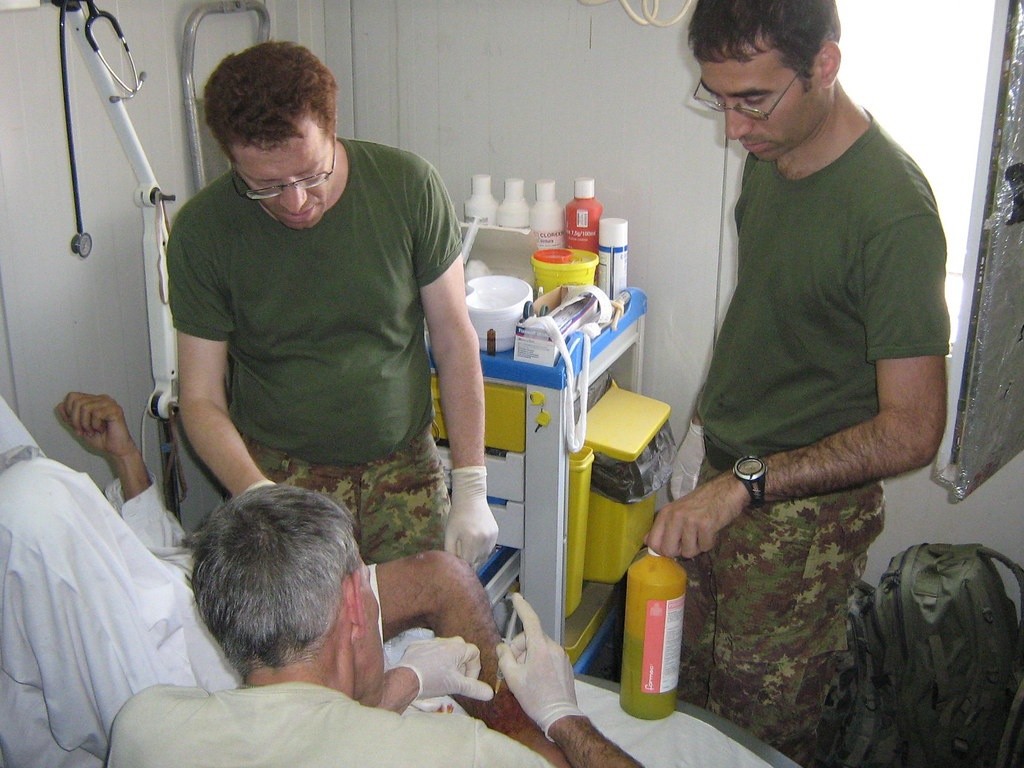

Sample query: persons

[643,1,951,765]
[0,391,575,768]
[166,41,499,567]
[105,484,642,768]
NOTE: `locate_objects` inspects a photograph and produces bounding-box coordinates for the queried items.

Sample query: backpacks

[814,543,1024,768]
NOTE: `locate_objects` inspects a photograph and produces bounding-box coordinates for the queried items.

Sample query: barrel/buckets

[530,248,599,299]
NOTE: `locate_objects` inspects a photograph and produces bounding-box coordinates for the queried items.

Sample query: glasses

[231,133,336,200]
[693,69,798,121]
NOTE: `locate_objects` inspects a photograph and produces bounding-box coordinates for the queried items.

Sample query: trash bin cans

[578,382,673,585]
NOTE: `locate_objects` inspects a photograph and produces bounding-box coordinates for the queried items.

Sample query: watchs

[732,453,768,508]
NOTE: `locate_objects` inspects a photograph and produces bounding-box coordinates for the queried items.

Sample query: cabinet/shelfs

[427,288,650,677]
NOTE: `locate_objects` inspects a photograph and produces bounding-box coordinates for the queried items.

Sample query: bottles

[496,178,529,228]
[464,175,499,226]
[530,179,566,250]
[566,178,603,287]
[620,547,687,720]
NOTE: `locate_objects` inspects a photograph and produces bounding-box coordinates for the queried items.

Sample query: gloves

[392,636,494,701]
[444,466,499,566]
[671,419,706,499]
[496,592,584,742]
[242,479,277,491]
